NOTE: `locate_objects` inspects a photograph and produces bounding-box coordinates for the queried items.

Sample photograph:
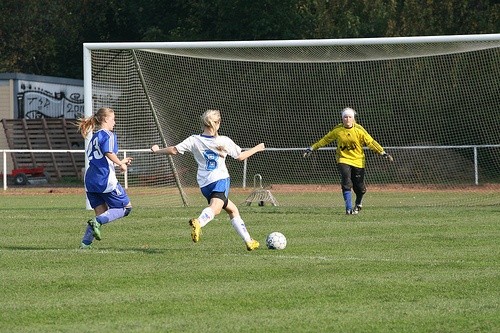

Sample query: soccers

[266,232,287,250]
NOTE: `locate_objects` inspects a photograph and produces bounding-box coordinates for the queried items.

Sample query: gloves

[383,152,393,161]
[303,147,312,158]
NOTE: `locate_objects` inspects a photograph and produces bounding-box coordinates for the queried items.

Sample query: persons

[303,109,394,215]
[75,108,134,245]
[151,110,264,251]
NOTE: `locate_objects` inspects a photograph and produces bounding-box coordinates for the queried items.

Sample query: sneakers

[87,218,102,242]
[247,238,260,252]
[189,218,201,243]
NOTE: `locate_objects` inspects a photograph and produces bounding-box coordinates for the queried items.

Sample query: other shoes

[346,204,363,215]
